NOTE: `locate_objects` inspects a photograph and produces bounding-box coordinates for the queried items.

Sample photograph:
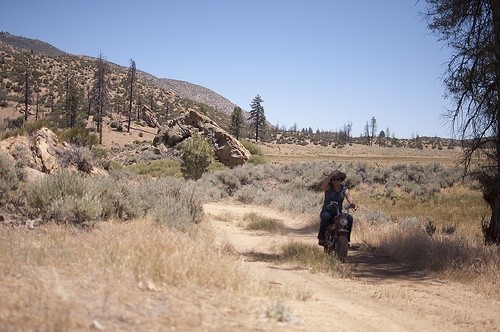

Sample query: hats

[330,170,347,184]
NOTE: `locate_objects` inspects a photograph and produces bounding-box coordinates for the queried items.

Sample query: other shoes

[318,238,326,245]
[347,242,351,247]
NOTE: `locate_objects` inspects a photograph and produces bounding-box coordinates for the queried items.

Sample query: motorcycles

[321,201,356,261]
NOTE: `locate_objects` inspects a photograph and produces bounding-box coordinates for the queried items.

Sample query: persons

[317,168,356,247]
